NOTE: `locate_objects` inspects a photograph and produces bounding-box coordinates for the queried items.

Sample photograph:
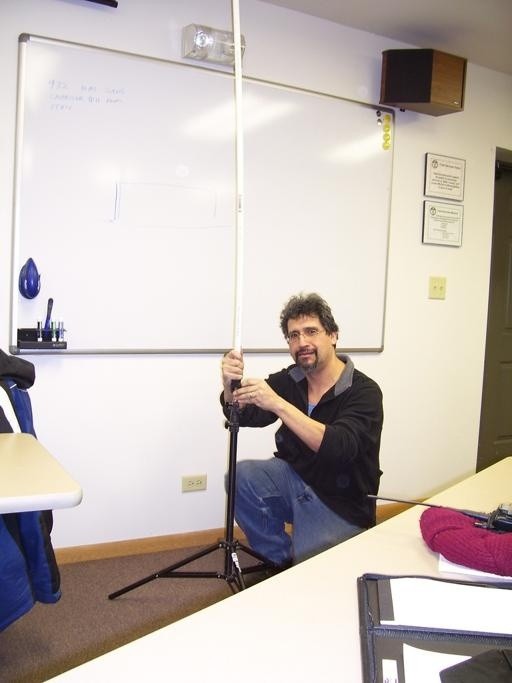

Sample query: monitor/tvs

[375,576,512,639]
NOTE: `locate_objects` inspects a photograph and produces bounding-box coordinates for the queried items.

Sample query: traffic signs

[182,473,208,493]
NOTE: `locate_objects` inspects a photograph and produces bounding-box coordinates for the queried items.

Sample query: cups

[285,326,328,345]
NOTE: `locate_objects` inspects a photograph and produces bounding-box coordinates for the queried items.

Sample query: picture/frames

[1,432,84,514]
[41,454,512,683]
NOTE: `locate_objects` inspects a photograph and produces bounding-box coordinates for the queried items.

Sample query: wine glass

[250,391,253,398]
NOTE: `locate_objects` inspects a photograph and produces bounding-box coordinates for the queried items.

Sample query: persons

[217,291,385,590]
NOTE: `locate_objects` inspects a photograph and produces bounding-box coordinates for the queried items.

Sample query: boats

[380,48,467,117]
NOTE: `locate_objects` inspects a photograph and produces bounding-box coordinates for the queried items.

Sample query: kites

[37,321,65,343]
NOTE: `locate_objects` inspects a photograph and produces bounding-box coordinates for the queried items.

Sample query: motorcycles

[108,377,282,598]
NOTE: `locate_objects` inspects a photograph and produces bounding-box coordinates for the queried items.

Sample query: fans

[8,33,396,354]
